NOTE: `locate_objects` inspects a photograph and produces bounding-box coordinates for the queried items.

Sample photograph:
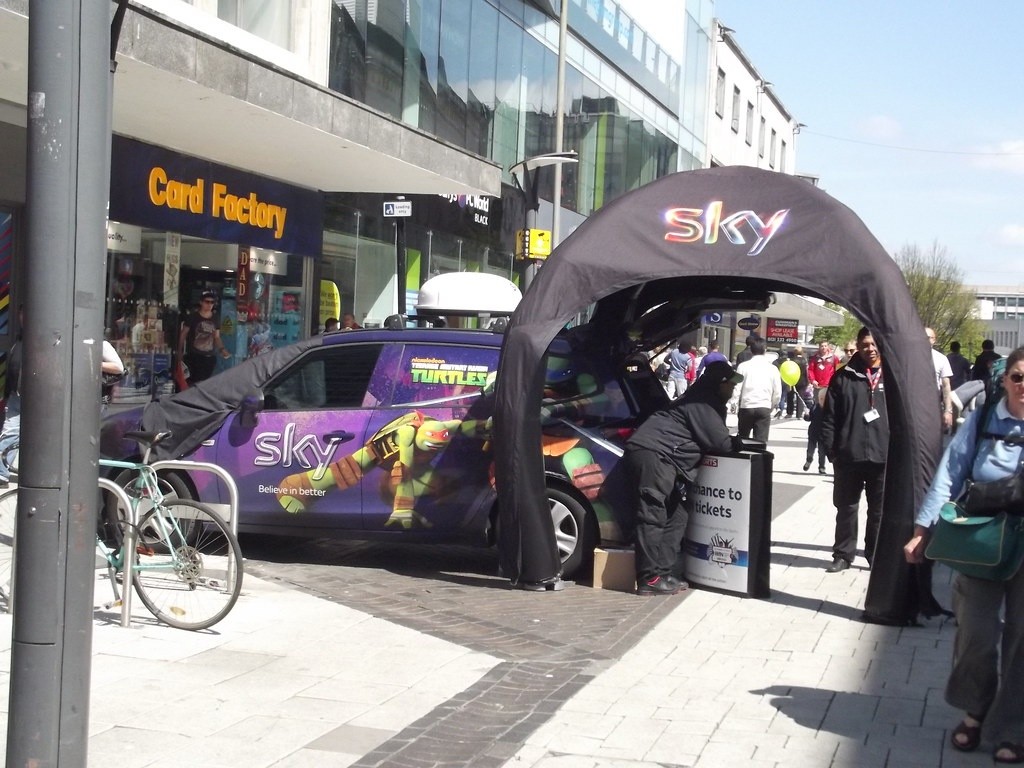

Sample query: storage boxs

[592,547,637,590]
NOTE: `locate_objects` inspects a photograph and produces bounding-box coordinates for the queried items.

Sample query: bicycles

[0,430,245,631]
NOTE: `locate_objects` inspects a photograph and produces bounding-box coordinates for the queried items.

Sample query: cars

[99,269,774,579]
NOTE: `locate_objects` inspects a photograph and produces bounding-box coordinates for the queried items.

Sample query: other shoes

[784,415,792,418]
[797,417,802,420]
[819,468,826,475]
[803,461,811,471]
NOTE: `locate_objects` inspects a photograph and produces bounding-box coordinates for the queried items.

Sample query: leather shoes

[828,558,851,571]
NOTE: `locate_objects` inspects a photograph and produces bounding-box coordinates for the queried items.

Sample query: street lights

[509,150,578,293]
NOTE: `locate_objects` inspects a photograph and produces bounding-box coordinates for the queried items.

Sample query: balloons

[780,360,801,387]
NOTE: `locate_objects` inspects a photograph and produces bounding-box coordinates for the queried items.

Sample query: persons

[639,328,1004,475]
[0,299,125,485]
[821,326,890,574]
[903,347,1024,763]
[625,361,744,595]
[323,312,447,332]
[180,290,231,388]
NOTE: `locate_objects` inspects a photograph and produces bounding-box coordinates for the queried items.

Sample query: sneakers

[636,575,689,596]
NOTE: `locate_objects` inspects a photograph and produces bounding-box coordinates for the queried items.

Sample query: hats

[706,361,744,384]
[325,317,339,328]
[201,290,215,299]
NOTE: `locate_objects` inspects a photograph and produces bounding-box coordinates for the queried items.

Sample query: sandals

[951,715,981,751]
[994,743,1023,762]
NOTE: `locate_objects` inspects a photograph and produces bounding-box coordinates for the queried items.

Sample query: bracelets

[219,347,225,351]
[944,408,954,413]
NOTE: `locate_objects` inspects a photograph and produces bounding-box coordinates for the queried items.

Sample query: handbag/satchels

[925,501,1015,580]
[655,363,670,381]
[967,474,1024,515]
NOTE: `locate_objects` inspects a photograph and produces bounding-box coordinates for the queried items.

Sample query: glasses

[1010,373,1024,383]
[203,299,214,304]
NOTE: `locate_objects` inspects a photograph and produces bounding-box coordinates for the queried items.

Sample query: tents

[495,164,947,629]
